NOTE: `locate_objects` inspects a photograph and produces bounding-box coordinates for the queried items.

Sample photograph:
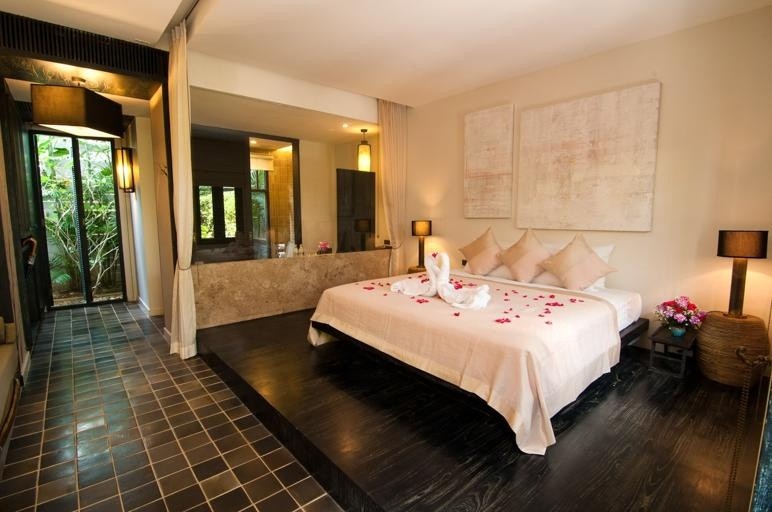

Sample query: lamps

[30,83,123,140]
[412,220,432,268]
[115,146,135,192]
[358,128,371,172]
[717,230,768,319]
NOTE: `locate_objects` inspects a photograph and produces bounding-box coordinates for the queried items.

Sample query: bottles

[292,244,304,257]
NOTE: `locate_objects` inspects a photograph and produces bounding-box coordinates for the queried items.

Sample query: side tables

[697,311,769,387]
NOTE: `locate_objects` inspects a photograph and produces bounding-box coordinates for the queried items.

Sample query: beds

[308,233,649,457]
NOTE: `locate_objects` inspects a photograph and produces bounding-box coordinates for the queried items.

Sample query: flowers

[655,296,704,329]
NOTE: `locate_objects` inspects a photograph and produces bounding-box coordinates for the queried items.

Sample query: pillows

[460,227,503,275]
[502,228,549,282]
[537,235,617,290]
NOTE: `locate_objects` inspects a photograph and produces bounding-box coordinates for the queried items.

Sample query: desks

[647,326,695,378]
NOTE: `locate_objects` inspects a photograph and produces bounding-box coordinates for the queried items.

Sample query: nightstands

[408,265,426,274]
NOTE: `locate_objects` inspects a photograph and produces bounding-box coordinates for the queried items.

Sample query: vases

[671,324,686,336]
